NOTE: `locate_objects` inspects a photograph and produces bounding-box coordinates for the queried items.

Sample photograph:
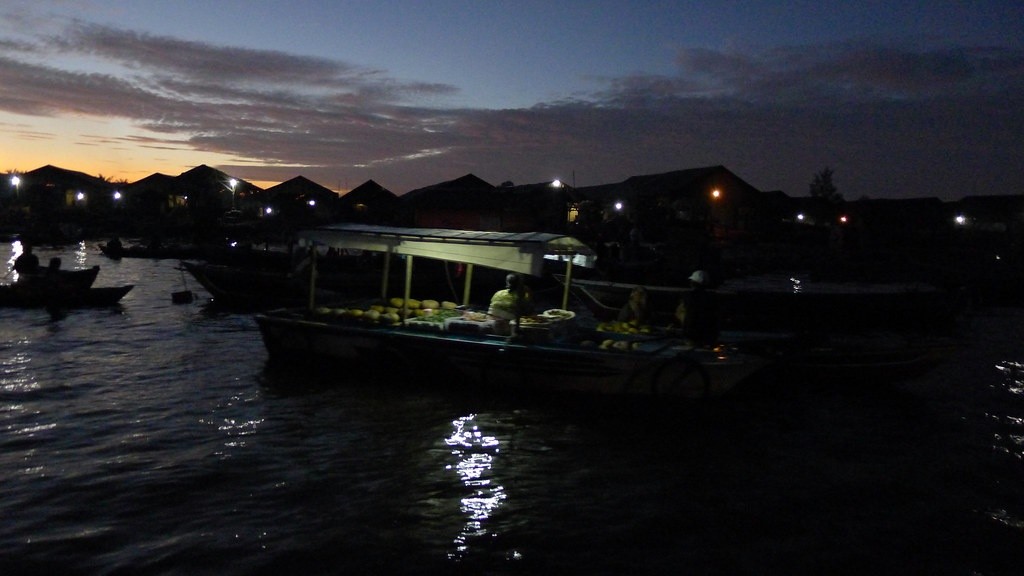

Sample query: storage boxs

[403,312,495,338]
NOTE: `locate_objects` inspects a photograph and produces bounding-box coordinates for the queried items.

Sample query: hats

[630,285,648,299]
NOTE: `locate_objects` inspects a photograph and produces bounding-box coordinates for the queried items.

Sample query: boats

[180,260,321,308]
[0,259,135,309]
[254,222,762,406]
[555,269,950,382]
[97,244,141,259]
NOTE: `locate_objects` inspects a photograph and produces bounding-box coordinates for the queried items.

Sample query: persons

[617,286,661,326]
[488,274,524,307]
[15,242,38,273]
[674,270,720,345]
